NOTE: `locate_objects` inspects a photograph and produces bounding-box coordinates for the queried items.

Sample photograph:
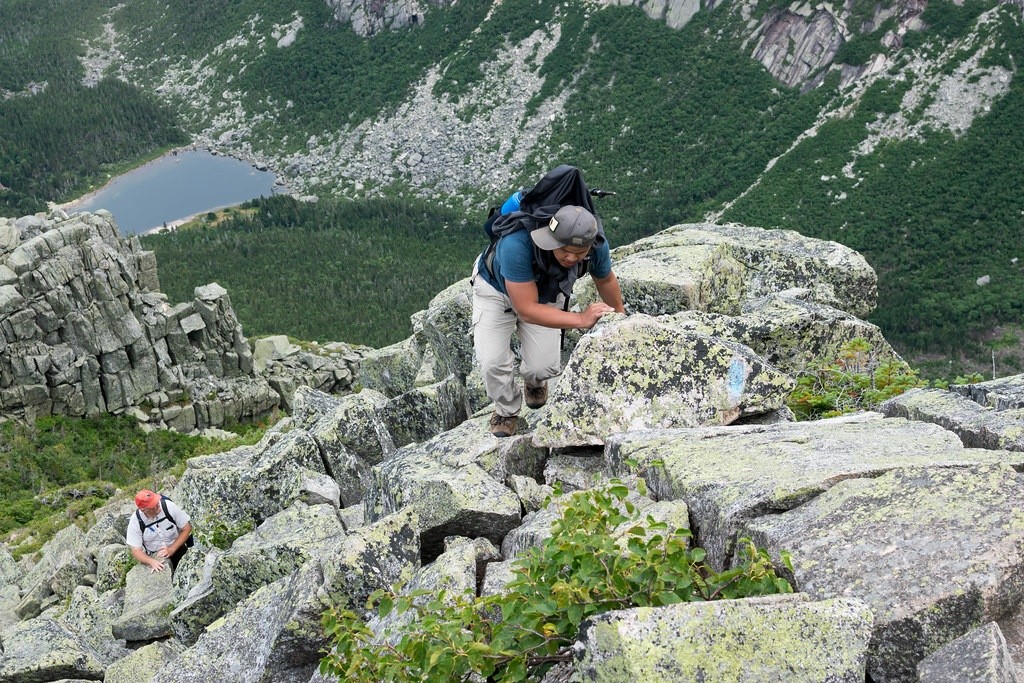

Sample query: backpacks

[485,167,603,279]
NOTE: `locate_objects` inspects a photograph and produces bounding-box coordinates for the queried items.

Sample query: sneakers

[524,380,549,409]
[489,412,517,436]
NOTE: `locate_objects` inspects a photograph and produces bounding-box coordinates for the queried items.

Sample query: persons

[470,206,625,437]
[126,489,194,574]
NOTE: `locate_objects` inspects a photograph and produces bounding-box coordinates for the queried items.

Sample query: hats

[136,490,162,509]
[530,204,598,251]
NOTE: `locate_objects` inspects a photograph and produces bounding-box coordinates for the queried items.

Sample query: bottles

[501,190,527,215]
